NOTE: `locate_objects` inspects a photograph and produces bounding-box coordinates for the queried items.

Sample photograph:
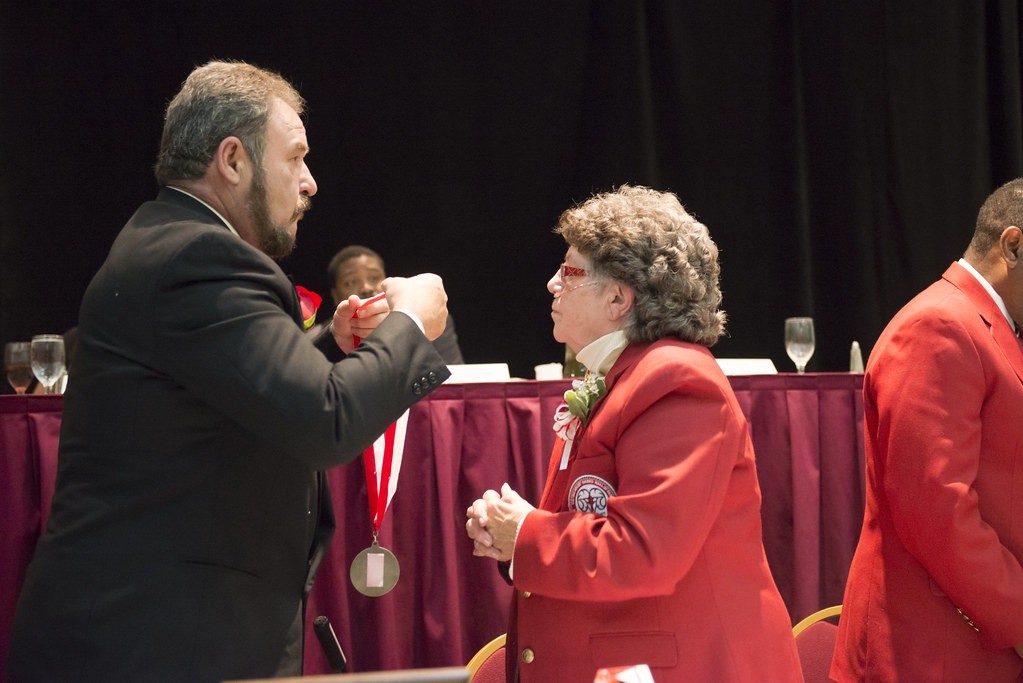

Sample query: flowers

[564,381,607,428]
[293,285,322,330]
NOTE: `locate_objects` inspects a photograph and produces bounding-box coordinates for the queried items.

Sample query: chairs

[792,604,844,683]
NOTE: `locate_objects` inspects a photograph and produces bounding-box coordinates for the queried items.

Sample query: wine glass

[4,334,65,393]
[785,318,816,373]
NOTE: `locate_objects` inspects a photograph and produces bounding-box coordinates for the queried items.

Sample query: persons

[329,246,463,365]
[828,178,1022,682]
[466,187,805,683]
[6,63,450,683]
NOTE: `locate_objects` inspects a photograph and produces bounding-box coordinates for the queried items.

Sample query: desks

[0,374,870,677]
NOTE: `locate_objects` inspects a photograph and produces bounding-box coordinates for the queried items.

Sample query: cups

[535,363,563,380]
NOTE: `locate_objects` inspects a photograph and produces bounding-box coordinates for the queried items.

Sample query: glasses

[560,264,593,281]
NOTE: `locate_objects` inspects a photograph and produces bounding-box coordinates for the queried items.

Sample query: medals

[349,547,399,597]
